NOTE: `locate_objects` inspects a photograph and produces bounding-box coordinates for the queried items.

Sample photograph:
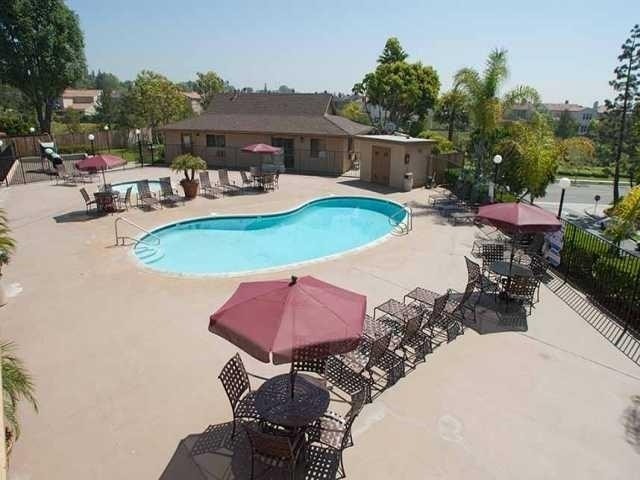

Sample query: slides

[44,148,70,179]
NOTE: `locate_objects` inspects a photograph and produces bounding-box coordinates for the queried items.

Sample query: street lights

[29,126,38,153]
[135,127,141,157]
[102,124,111,154]
[553,177,572,221]
[489,153,504,204]
[88,132,95,154]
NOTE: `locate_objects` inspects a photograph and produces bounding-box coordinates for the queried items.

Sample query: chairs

[218,343,366,480]
[428,185,485,226]
[357,288,463,390]
[78,166,282,217]
[462,229,553,320]
[53,163,102,186]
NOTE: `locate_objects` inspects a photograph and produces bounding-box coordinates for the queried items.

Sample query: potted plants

[169,151,208,201]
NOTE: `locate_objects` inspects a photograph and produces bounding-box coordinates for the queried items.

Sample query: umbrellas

[208,275,367,401]
[475,198,562,272]
[76,153,127,192]
[240,143,281,176]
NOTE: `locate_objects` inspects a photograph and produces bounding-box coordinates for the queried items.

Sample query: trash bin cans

[403,173,413,192]
